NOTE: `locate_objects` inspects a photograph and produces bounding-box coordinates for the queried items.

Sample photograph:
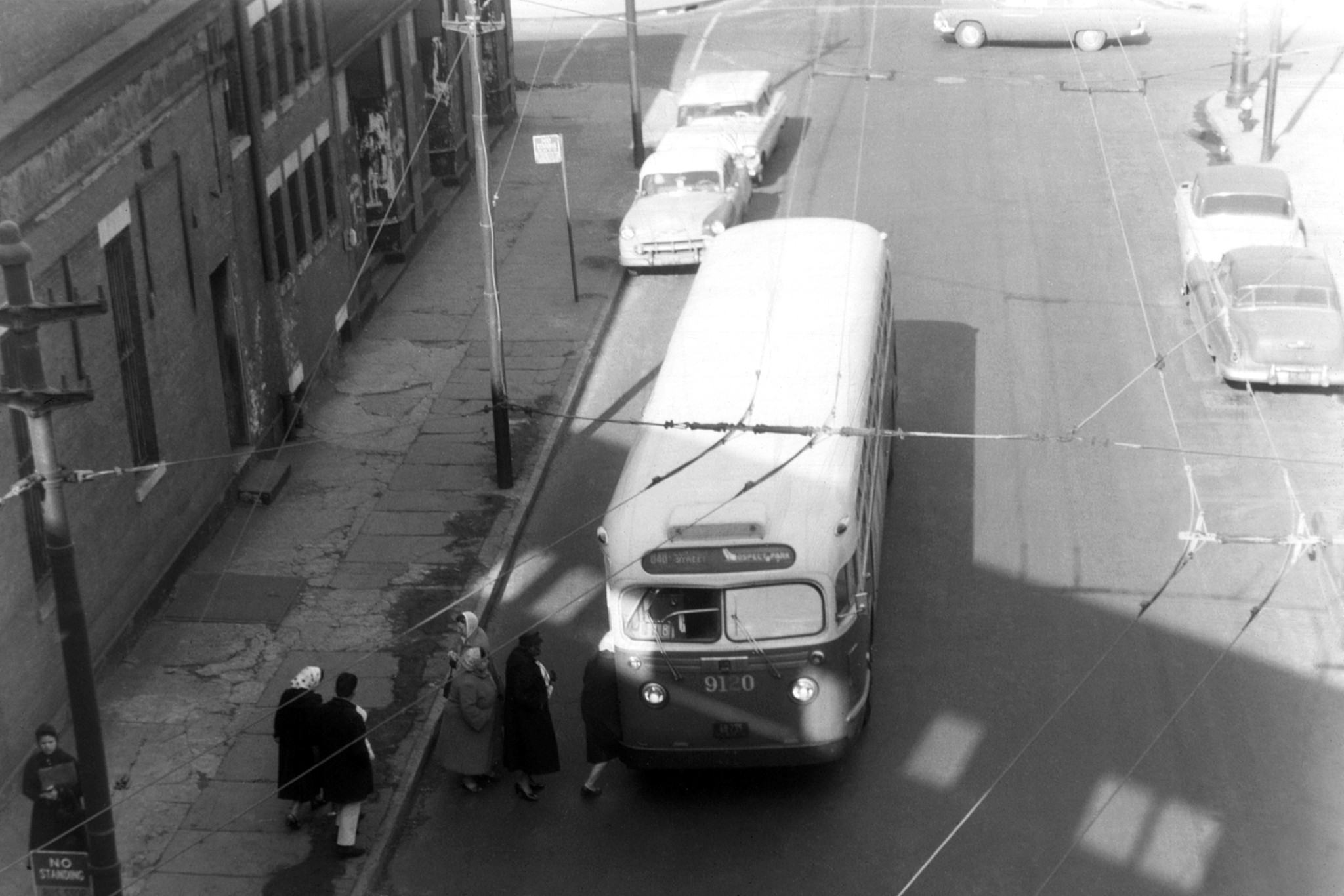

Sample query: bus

[594,213,898,799]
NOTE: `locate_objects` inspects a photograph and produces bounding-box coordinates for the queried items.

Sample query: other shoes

[581,784,602,797]
[515,783,543,801]
[461,779,483,793]
[337,844,366,859]
[527,778,545,791]
[286,814,302,831]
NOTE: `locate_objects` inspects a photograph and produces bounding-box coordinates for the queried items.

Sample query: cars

[617,143,742,274]
[933,1,1148,52]
[1180,242,1344,392]
[655,70,786,187]
[1175,163,1307,265]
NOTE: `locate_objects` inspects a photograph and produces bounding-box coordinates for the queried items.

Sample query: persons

[305,672,375,857]
[499,633,561,801]
[448,611,501,713]
[24,724,89,870]
[437,647,510,794]
[272,667,324,831]
